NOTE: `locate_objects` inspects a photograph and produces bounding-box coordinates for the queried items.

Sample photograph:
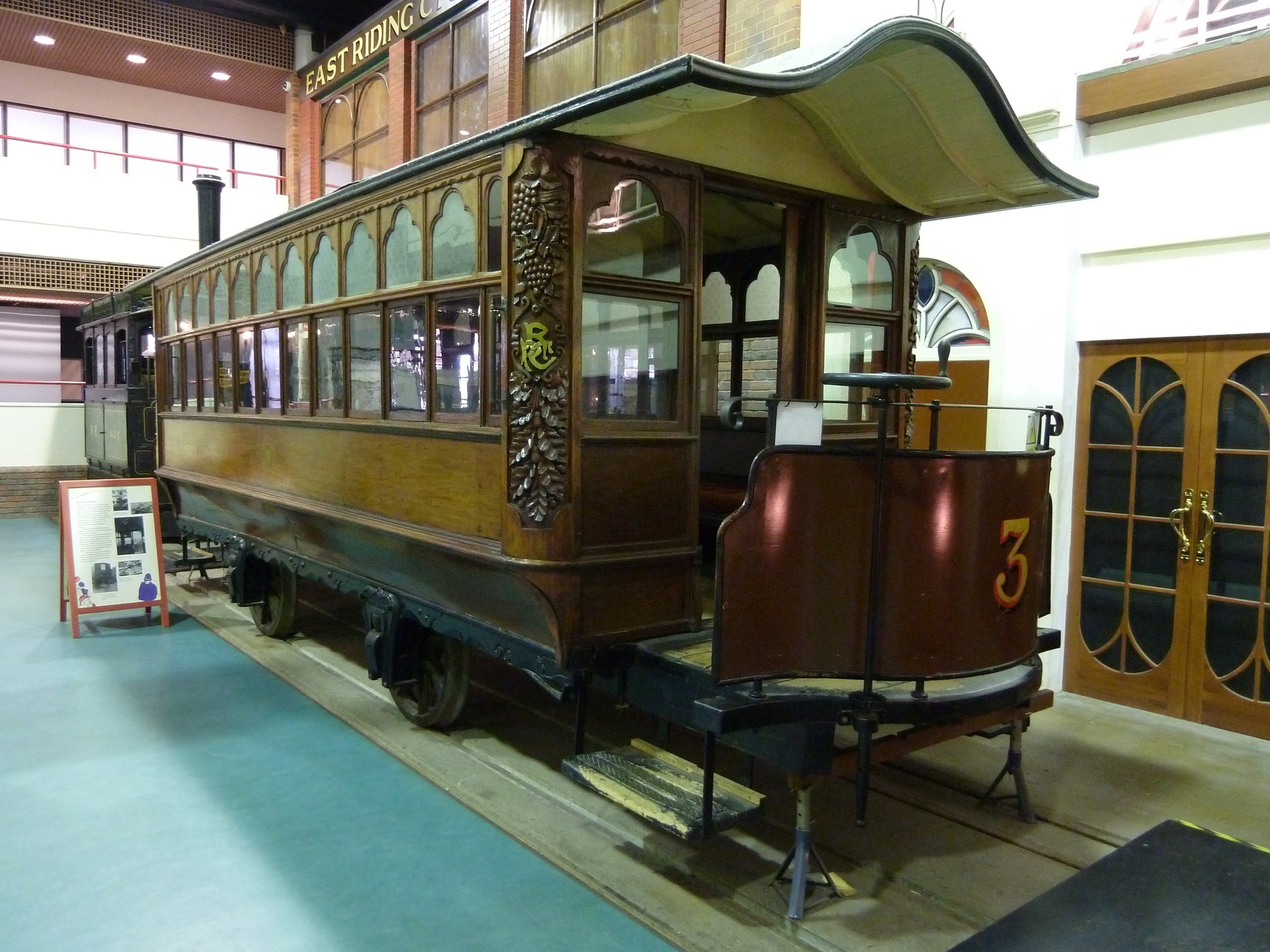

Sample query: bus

[74,11,1100,838]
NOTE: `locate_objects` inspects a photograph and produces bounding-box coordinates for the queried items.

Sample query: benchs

[698,470,747,526]
